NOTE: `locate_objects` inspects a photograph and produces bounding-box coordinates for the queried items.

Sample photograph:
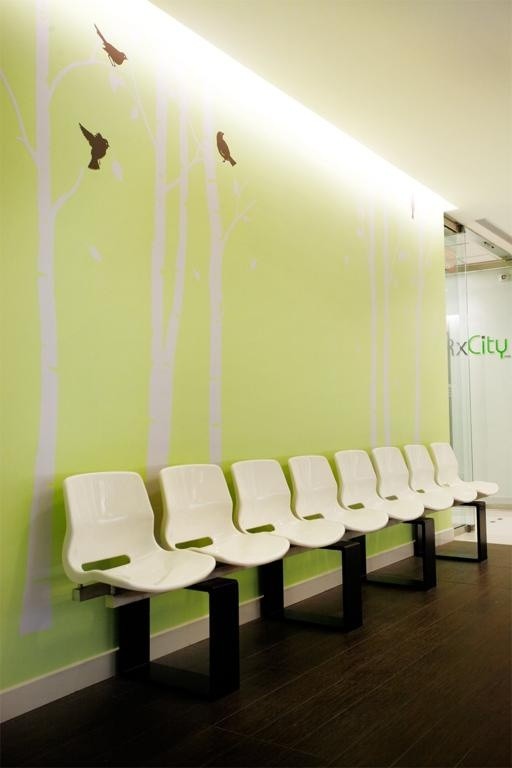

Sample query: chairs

[62,440,500,693]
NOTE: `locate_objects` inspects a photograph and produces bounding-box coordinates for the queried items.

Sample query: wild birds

[93,23,128,66]
[79,123,109,169]
[217,132,235,166]
